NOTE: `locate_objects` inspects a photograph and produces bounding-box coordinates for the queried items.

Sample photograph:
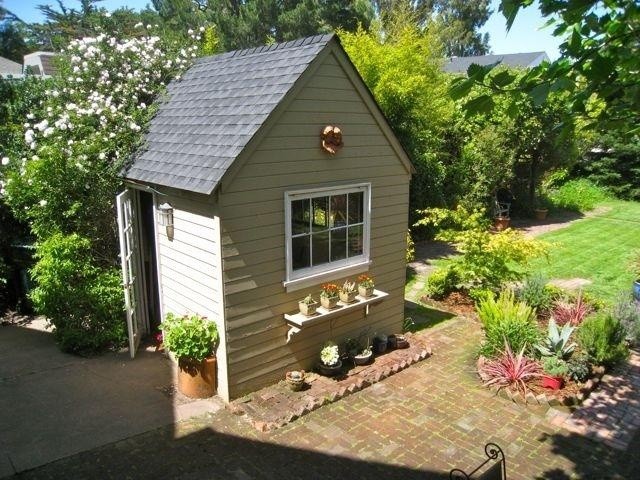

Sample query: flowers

[318,281,339,298]
[357,274,374,289]
[319,339,340,366]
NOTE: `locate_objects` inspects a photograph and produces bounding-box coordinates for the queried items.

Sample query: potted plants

[632,248,640,302]
[348,332,373,364]
[300,293,318,317]
[543,355,569,391]
[337,280,357,304]
[159,310,221,400]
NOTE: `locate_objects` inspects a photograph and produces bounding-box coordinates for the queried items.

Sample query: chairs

[449,442,508,480]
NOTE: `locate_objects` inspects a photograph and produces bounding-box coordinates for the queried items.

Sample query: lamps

[157,201,174,227]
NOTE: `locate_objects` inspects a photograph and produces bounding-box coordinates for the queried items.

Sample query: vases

[534,208,548,221]
[374,335,388,353]
[389,333,406,349]
[318,360,343,376]
[286,370,306,392]
[492,216,511,234]
[358,286,374,298]
[320,296,338,311]
